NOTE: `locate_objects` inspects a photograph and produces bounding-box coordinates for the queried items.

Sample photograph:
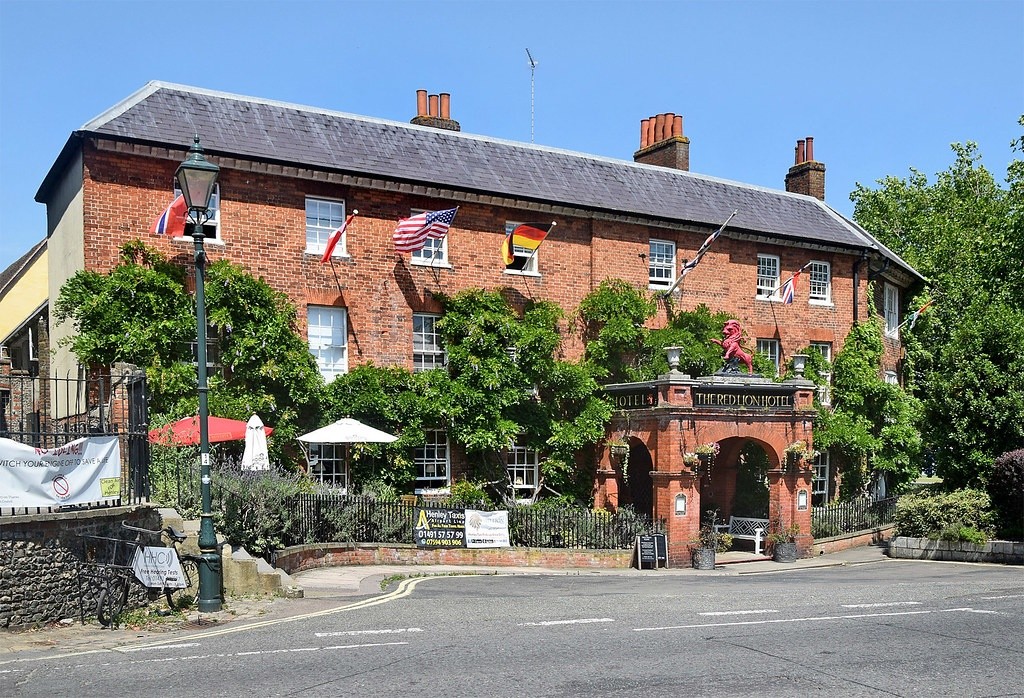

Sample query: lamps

[316,463,326,484]
[513,477,523,494]
[426,465,435,488]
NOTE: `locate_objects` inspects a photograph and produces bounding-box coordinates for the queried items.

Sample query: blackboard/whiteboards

[633,534,658,562]
[650,533,668,560]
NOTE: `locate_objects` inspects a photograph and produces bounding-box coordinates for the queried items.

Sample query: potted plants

[604,437,630,484]
[765,516,802,563]
[683,445,718,483]
[784,439,820,475]
[685,510,733,569]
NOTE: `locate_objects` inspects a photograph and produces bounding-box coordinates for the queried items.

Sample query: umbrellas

[146,410,275,448]
[240,411,270,471]
[295,414,399,495]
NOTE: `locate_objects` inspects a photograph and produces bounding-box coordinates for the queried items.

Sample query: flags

[392,207,458,253]
[501,221,554,266]
[149,194,189,237]
[781,267,805,304]
[320,215,354,262]
[908,301,931,331]
[681,213,735,276]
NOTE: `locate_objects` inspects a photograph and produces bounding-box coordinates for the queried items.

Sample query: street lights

[172,130,227,614]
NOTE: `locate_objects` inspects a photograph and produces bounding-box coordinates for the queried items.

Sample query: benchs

[715,515,769,555]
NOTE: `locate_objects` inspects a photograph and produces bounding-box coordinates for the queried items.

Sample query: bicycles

[96,518,200,627]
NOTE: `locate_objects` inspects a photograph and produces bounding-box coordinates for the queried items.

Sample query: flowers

[710,442,721,457]
